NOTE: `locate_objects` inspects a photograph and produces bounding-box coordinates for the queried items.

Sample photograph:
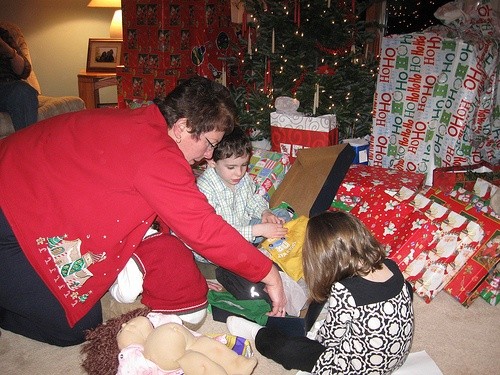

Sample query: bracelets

[8,48,17,59]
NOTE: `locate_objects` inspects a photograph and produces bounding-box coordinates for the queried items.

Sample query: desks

[76,72,117,110]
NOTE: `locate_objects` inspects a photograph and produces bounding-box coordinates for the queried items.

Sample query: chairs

[0,21,87,137]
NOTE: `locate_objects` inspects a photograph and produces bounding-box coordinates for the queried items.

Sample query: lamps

[87,0,122,39]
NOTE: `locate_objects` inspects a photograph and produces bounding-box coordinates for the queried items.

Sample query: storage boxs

[115,0,500,311]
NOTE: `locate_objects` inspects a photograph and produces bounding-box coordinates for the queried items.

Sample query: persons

[226,210,414,375]
[108,220,208,331]
[79,307,257,375]
[171,121,296,263]
[0,76,287,347]
[0,27,39,133]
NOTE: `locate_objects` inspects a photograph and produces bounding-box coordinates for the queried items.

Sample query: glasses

[201,132,217,151]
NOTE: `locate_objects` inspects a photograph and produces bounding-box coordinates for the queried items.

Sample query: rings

[278,310,283,313]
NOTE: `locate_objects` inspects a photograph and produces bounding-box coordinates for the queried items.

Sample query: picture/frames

[86,38,123,73]
